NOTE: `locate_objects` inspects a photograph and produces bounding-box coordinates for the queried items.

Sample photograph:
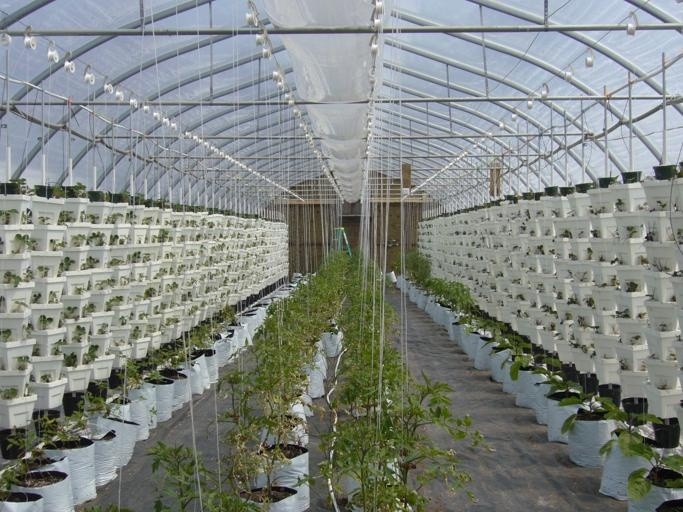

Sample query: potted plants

[145,239,500,510]
[393,162,683,512]
[2,162,319,512]
